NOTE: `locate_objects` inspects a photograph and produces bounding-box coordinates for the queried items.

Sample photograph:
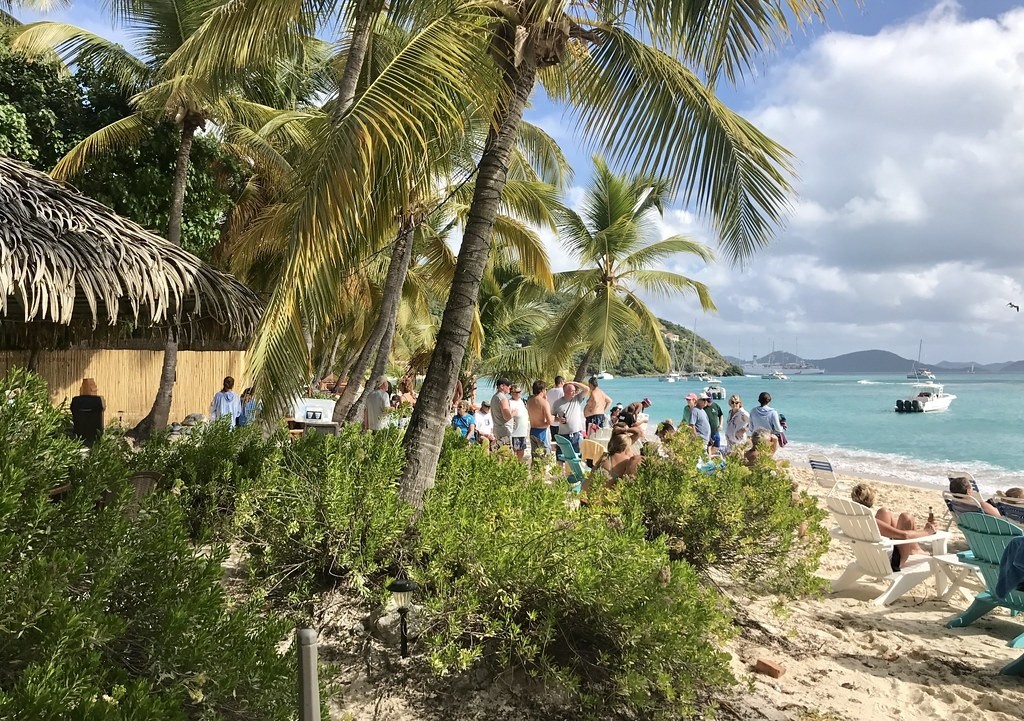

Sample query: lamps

[386,580,418,613]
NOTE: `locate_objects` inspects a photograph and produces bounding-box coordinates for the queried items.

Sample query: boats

[592,369,614,380]
[895,380,957,414]
[743,336,825,375]
[761,368,788,380]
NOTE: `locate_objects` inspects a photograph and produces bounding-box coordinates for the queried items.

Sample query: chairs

[554,427,644,483]
[942,491,985,533]
[992,494,1024,533]
[942,469,984,517]
[943,511,1024,648]
[96,472,165,511]
[806,452,854,501]
[823,494,952,607]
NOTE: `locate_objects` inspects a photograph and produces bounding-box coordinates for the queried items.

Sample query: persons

[209,376,241,432]
[850,483,936,572]
[744,427,817,542]
[683,392,785,466]
[364,374,495,452]
[1006,488,1024,505]
[949,477,1002,518]
[236,387,263,427]
[491,375,676,508]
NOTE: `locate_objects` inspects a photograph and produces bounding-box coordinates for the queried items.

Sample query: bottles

[562,410,566,424]
[928,507,936,534]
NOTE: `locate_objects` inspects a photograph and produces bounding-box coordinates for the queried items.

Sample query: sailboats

[657,319,722,384]
[907,338,936,380]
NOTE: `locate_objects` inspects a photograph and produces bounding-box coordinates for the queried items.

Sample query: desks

[934,553,989,604]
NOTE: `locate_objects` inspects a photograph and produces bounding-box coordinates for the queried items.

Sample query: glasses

[513,391,522,394]
[731,401,739,404]
[702,399,709,401]
[459,406,463,409]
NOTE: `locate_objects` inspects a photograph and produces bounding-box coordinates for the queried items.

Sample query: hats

[617,405,623,409]
[497,378,512,385]
[684,393,698,401]
[665,419,674,426]
[698,393,711,399]
[644,397,652,407]
[482,401,491,407]
[375,376,387,389]
